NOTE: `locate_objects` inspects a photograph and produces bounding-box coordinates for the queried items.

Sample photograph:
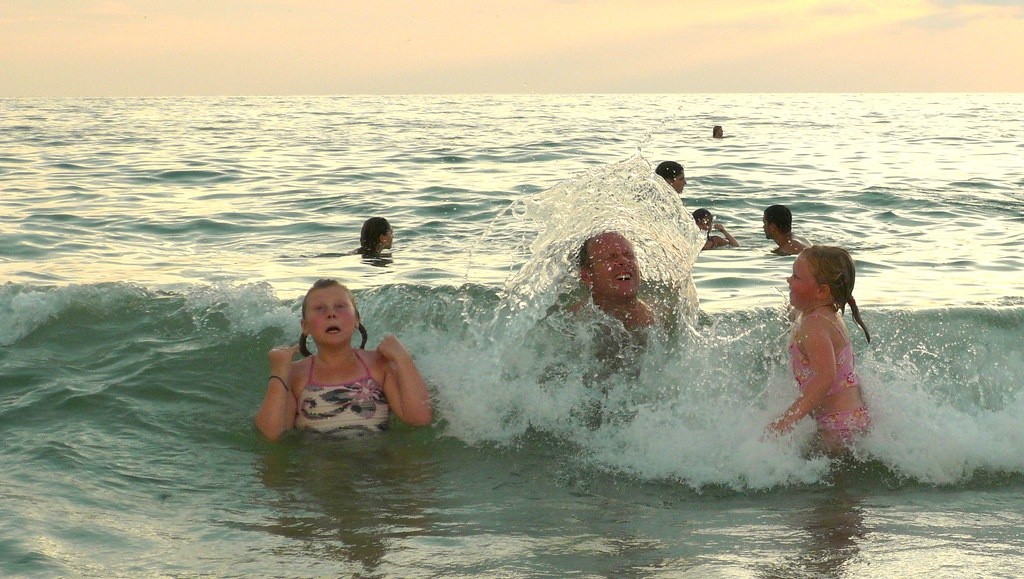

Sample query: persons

[255,125,872,452]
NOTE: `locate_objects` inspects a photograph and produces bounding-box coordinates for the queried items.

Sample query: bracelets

[269,375,289,391]
